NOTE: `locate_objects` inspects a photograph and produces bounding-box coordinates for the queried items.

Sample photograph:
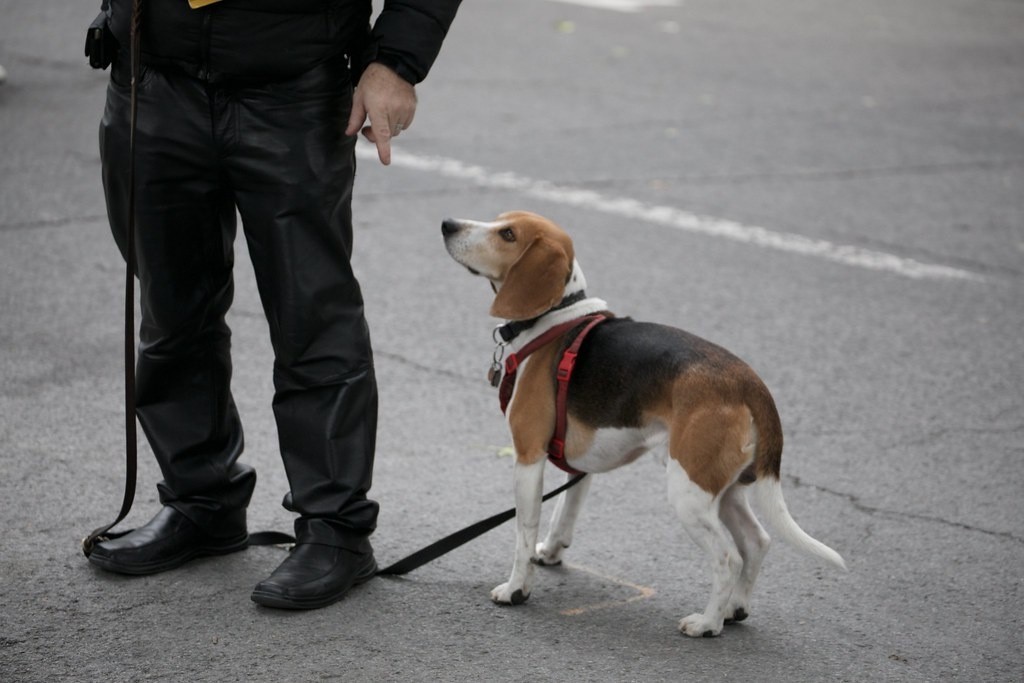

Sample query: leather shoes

[88,504,251,576]
[250,542,378,610]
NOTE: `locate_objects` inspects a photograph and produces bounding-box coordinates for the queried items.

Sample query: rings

[395,123,404,129]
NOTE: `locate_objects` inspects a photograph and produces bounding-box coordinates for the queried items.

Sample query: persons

[86,0,461,612]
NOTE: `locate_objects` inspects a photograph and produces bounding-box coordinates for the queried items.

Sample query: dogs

[441,210,846,637]
